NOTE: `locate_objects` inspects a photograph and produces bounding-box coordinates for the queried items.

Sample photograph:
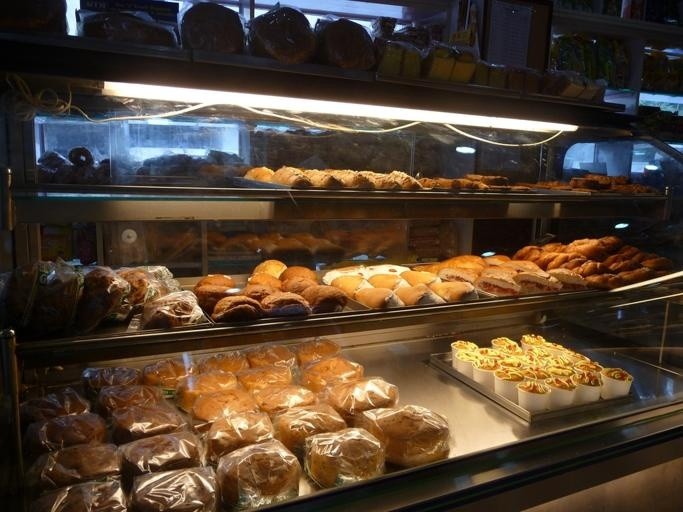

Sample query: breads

[2,236,683,327]
[1,0,682,114]
[28,335,452,511]
[33,145,664,197]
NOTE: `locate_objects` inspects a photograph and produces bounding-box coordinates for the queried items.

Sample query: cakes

[450,333,635,413]
